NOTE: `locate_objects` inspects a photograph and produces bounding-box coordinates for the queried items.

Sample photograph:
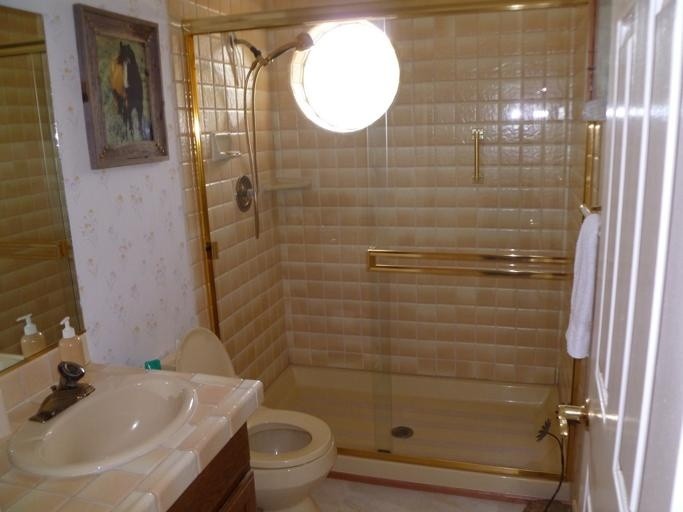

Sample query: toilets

[161,325,337,511]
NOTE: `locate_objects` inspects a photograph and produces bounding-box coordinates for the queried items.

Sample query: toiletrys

[58,315,87,372]
[16,312,47,359]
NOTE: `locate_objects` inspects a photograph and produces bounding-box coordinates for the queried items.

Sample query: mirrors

[0,5,86,377]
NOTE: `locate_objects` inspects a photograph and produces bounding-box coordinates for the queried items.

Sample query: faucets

[27,388,78,424]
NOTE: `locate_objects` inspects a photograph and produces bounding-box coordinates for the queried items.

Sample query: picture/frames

[75,2,169,168]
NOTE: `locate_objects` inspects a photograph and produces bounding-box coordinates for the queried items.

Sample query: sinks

[6,370,199,477]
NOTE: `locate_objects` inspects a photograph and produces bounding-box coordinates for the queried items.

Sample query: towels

[565,213,602,358]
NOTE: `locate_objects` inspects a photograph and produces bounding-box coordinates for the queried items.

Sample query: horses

[109,41,146,143]
